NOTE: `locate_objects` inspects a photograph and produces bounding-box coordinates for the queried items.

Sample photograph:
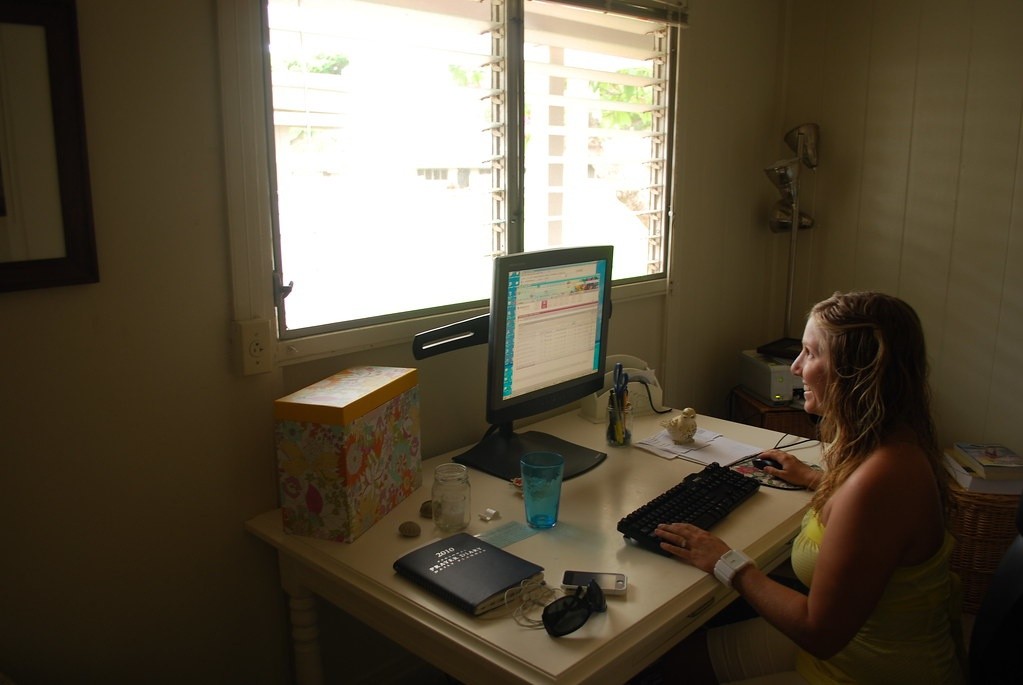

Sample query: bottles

[432,463,472,534]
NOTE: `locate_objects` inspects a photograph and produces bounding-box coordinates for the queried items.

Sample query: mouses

[752,458,783,471]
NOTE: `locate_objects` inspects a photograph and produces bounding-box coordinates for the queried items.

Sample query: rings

[682,540,689,548]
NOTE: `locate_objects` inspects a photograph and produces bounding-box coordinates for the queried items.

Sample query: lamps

[758,120,819,360]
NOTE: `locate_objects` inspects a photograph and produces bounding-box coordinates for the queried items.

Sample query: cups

[605,404,634,449]
[520,450,565,528]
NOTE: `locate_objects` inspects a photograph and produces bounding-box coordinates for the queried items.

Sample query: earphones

[522,592,533,608]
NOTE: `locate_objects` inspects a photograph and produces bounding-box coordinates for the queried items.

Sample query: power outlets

[231,318,274,377]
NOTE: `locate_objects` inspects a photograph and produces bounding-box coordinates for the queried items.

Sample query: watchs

[713,548,759,587]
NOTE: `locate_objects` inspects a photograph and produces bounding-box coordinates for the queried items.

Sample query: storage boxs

[274,365,424,541]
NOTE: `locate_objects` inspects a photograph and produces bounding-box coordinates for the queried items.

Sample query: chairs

[948,490,1023,685]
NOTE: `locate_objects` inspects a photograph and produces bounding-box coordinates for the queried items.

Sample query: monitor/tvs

[452,246,615,484]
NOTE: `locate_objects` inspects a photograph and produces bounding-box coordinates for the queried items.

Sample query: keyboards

[616,462,760,558]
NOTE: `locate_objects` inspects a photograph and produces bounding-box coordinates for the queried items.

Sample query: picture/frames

[0,0,100,288]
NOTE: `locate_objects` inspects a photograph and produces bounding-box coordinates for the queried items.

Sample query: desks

[247,407,842,685]
[730,388,822,442]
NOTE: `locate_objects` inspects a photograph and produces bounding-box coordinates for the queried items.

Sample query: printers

[740,349,806,410]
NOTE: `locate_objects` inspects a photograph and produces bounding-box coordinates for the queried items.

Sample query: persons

[653,291,964,685]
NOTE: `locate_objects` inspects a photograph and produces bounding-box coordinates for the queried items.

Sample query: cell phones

[561,570,627,592]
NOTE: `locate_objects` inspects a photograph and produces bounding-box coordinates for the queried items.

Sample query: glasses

[542,578,607,637]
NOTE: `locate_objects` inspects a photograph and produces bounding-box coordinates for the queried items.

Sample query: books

[392,532,547,617]
[942,440,1023,495]
